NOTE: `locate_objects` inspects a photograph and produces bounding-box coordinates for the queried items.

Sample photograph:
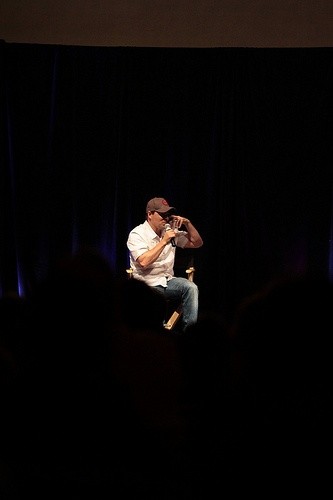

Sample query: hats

[146,198,176,214]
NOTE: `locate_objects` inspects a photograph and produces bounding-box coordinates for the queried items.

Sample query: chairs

[128,267,195,330]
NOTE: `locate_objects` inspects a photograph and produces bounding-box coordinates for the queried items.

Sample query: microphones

[165,224,176,247]
